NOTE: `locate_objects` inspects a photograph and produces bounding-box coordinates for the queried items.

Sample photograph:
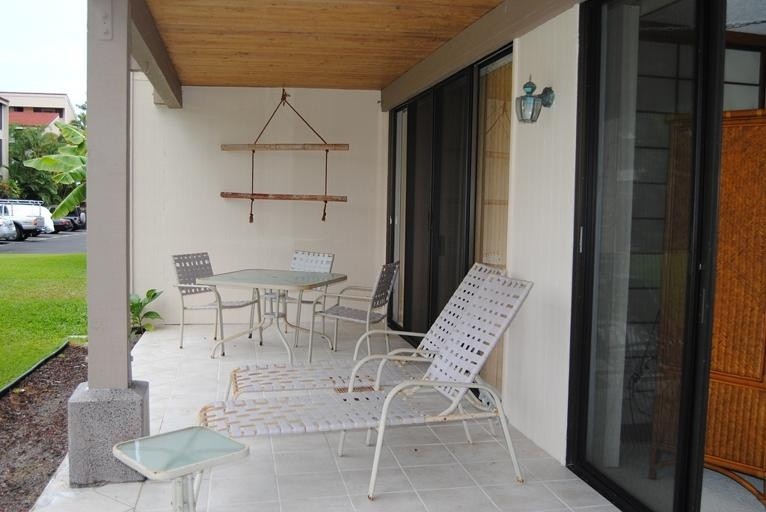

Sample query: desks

[112,426,248,512]
[195,269,347,369]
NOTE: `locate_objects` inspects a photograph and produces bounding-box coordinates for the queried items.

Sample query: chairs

[223,263,506,402]
[283,249,335,347]
[174,253,264,357]
[306,259,401,367]
[195,272,536,502]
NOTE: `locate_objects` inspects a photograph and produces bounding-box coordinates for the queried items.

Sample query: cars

[0,199,87,241]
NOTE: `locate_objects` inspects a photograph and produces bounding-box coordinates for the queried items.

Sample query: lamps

[515,73,554,124]
